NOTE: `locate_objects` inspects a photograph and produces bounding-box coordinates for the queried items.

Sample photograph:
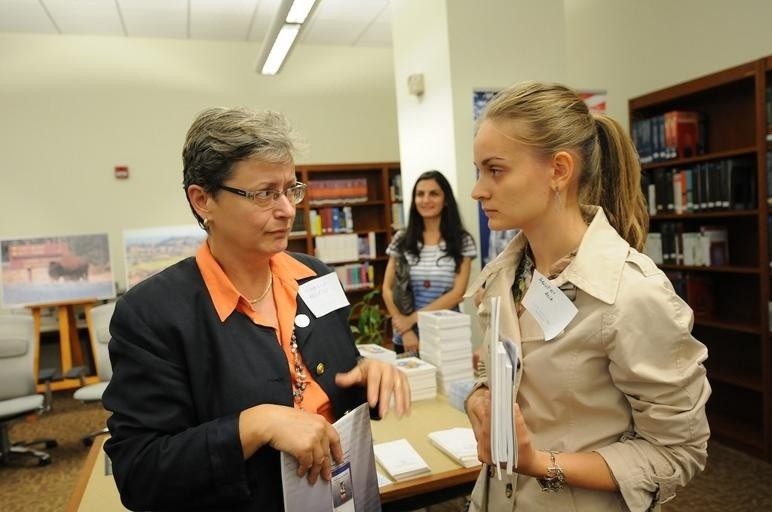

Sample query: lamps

[0,308,59,467]
[68,299,117,446]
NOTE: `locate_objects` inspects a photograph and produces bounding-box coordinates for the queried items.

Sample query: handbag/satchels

[538,450,566,497]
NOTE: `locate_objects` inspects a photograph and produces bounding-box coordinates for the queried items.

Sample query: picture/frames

[67,396,482,512]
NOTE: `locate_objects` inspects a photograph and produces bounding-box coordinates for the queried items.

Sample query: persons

[382,170,476,355]
[463,81,714,512]
[102,107,411,510]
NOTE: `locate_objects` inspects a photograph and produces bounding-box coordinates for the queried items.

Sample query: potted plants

[124,224,206,294]
[0,231,117,309]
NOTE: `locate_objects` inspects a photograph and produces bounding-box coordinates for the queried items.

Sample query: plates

[627,57,771,462]
[283,162,403,349]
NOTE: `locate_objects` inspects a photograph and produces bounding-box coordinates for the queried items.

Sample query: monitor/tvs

[308,207,354,234]
[304,178,368,204]
[389,173,403,201]
[357,342,396,409]
[427,427,482,468]
[447,378,477,412]
[415,309,475,396]
[638,160,754,215]
[639,222,729,269]
[632,111,714,165]
[489,296,521,480]
[279,404,383,512]
[313,231,378,264]
[395,357,438,403]
[328,262,375,291]
[392,202,404,231]
[659,268,712,315]
[371,439,431,481]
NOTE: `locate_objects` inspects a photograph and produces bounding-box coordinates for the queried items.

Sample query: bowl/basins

[248,266,274,304]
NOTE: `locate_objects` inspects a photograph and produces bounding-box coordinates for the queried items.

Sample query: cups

[213,181,309,207]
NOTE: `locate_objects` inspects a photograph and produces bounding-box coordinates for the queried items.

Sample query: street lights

[393,232,415,315]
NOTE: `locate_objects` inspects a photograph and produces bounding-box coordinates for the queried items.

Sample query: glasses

[260,25,302,76]
[283,0,317,25]
